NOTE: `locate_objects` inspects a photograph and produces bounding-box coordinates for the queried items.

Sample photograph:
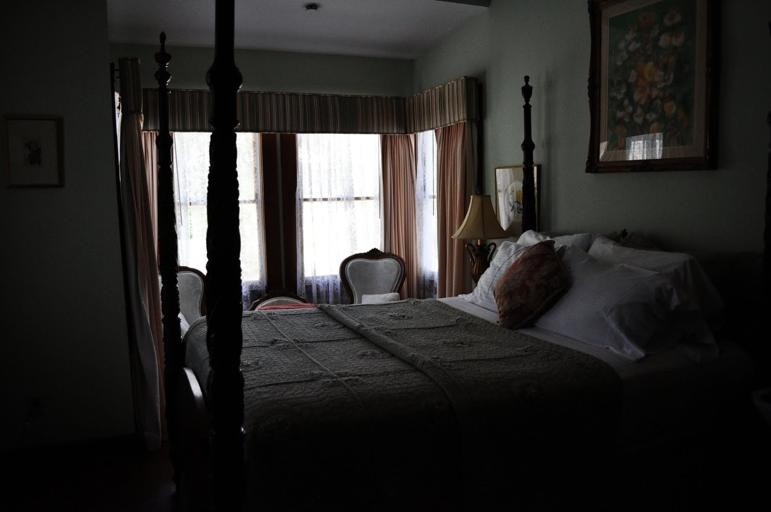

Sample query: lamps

[454,193,507,283]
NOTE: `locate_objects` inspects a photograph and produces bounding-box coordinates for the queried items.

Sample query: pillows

[463,229,695,361]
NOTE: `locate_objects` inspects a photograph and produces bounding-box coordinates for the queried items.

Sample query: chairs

[342,247,406,304]
[159,266,206,326]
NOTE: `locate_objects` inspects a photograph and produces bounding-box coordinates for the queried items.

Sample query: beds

[162,230,771,510]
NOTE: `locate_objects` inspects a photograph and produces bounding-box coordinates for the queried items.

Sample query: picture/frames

[587,1,722,172]
[496,166,540,233]
[5,113,64,189]
